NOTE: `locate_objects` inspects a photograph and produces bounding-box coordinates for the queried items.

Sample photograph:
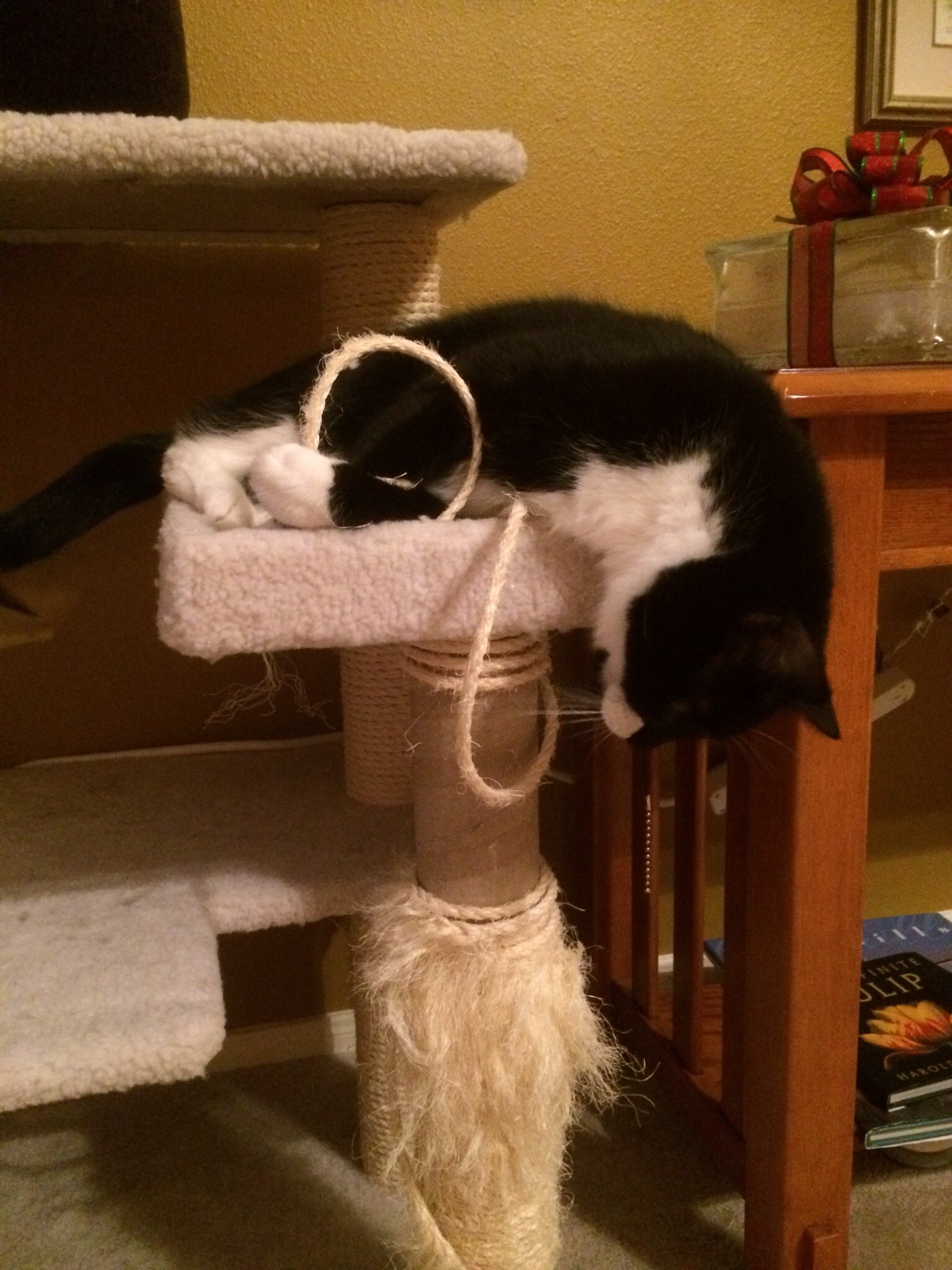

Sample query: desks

[585,366,952,1269]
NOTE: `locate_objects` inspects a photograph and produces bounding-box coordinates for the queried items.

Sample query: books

[705,906,952,1107]
[842,1089,952,1153]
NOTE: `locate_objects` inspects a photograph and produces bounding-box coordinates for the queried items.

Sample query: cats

[0,293,843,752]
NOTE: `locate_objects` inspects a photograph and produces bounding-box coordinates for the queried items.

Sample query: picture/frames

[861,1,952,135]
